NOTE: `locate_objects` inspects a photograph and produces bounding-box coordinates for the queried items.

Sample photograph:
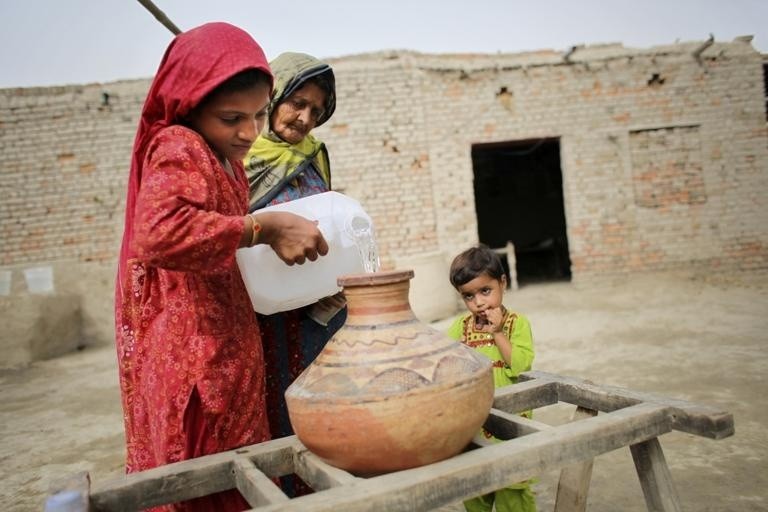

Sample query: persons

[114,21,330,512]
[443,246,535,511]
[233,50,348,501]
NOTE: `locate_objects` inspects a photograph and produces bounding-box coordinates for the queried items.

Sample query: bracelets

[244,213,261,247]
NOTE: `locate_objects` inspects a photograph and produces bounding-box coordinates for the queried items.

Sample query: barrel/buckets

[235,189,380,316]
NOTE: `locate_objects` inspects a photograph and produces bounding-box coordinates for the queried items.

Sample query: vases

[285,269,496,476]
